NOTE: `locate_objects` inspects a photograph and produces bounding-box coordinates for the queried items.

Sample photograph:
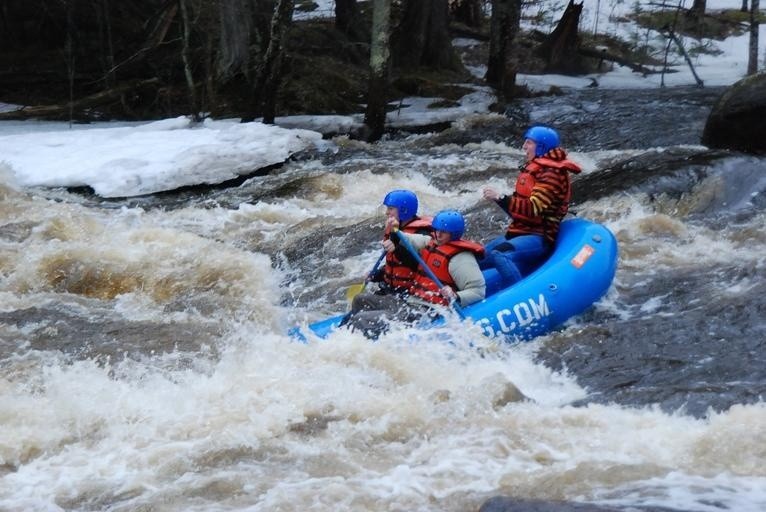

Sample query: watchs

[499,194,507,200]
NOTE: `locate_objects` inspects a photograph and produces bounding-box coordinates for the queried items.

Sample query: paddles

[347,247,388,300]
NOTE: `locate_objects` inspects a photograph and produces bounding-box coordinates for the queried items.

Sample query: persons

[353,210,486,342]
[472,126,571,287]
[338,190,436,327]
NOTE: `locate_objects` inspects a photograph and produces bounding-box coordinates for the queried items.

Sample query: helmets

[431,209,465,241]
[383,190,418,222]
[524,125,561,157]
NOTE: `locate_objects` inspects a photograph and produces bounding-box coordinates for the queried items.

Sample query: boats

[285,216,618,357]
